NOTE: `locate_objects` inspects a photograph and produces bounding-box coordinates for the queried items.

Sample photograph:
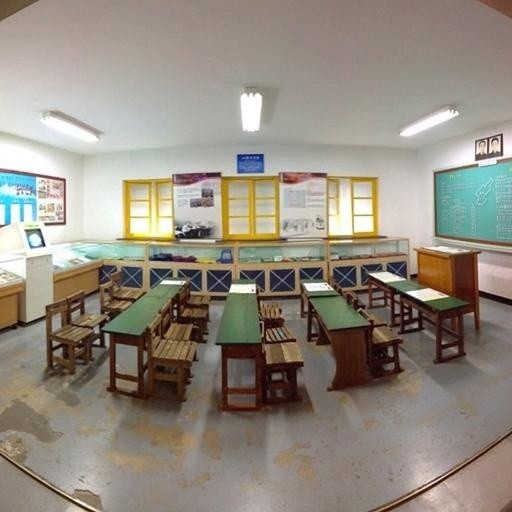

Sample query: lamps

[395,104,460,137]
[240,85,262,134]
[40,110,103,144]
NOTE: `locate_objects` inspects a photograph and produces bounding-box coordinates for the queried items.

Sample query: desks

[398,284,472,365]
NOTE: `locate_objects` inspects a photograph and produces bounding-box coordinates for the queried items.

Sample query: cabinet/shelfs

[82,236,411,298]
[0,242,103,333]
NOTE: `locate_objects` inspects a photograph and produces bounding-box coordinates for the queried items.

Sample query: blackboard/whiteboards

[430,155,512,247]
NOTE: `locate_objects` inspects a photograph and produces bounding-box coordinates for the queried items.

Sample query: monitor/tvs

[25,229,46,248]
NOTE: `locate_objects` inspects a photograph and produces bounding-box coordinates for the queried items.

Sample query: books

[339,254,369,259]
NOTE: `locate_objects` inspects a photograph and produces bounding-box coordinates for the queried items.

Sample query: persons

[489,138,501,157]
[475,141,487,160]
[182,219,214,237]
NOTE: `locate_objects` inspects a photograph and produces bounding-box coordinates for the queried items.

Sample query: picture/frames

[474,133,503,163]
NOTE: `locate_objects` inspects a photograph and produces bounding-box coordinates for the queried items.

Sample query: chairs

[47,298,94,374]
[216,270,415,412]
[99,273,210,404]
[66,289,108,359]
